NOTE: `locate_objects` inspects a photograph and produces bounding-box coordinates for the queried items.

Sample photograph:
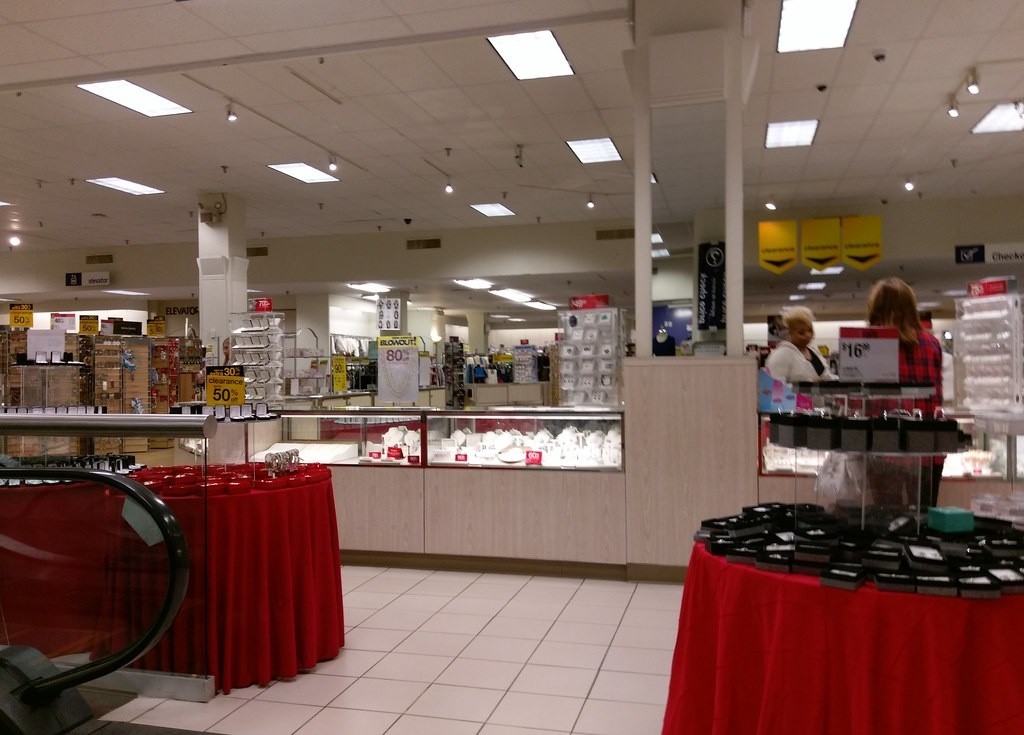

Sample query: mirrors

[647,98,730,358]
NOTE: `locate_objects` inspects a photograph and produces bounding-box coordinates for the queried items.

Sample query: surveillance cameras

[874,54,886,62]
[404,219,411,224]
[818,84,827,91]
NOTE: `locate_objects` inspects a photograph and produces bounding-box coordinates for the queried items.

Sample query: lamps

[965,66,979,95]
[445,176,453,193]
[904,175,913,191]
[947,91,959,118]
[587,193,594,208]
[765,194,777,210]
[225,98,238,121]
[328,152,337,171]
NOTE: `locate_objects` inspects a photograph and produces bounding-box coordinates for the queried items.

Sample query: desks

[0,466,149,656]
[661,541,1024,735]
[94,479,346,699]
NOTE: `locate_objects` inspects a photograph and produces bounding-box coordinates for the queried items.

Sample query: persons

[867,277,946,513]
[767,314,789,337]
[764,305,838,381]
[652,328,675,357]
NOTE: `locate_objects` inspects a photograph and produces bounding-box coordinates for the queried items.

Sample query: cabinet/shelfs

[952,290,1024,408]
[1,308,628,466]
